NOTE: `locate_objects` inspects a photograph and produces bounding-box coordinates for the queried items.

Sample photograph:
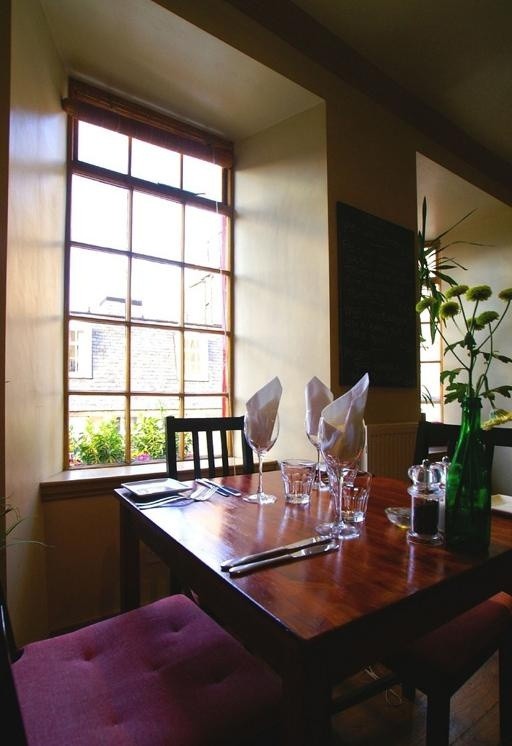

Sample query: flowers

[418,275,511,513]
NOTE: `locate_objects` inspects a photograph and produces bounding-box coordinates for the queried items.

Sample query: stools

[321,587,512,746]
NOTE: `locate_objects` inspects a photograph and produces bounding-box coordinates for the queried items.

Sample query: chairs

[163,410,279,645]
[0,585,280,746]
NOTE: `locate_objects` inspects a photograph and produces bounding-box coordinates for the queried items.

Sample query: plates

[489,493,512,516]
[120,476,194,500]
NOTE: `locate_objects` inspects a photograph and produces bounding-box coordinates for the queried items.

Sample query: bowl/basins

[384,506,410,530]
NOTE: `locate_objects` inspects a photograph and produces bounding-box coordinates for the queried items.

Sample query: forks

[134,486,208,507]
[139,486,220,510]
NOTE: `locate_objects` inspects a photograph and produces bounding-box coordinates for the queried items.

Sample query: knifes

[202,477,242,497]
[195,479,230,497]
[228,542,340,578]
[219,535,334,571]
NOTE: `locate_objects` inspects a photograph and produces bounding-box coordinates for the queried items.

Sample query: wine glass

[314,417,368,544]
[239,411,281,507]
[304,413,334,493]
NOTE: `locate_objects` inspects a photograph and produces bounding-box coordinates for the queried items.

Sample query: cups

[330,468,375,524]
[403,455,452,548]
[282,457,313,506]
[328,460,360,498]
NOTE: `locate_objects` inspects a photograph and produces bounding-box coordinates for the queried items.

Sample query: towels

[306,370,371,467]
[242,375,282,448]
